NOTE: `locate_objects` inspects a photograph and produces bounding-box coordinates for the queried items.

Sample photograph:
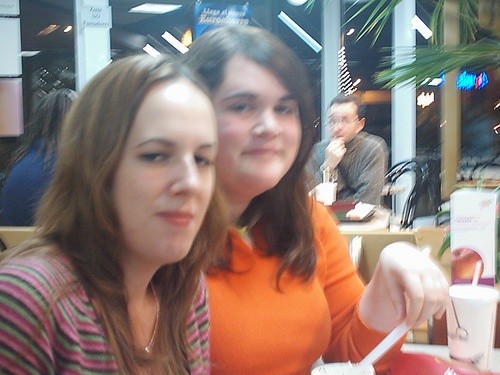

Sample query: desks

[339,214,450,343]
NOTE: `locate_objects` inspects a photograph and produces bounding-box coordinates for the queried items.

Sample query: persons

[1,85,83,226]
[171,24,451,375]
[303,91,388,211]
[1,52,231,375]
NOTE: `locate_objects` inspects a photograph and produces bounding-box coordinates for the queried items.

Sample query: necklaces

[137,277,158,354]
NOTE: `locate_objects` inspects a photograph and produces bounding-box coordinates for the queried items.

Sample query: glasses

[328,115,360,126]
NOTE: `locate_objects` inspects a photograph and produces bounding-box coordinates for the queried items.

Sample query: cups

[311,363,375,375]
[445,285,499,372]
